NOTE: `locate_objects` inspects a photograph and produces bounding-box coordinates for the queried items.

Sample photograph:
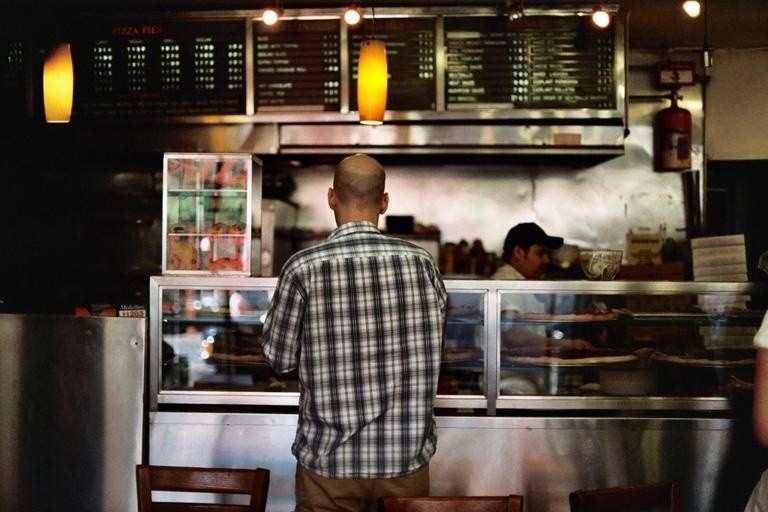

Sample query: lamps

[356,8,389,123]
[262,8,283,26]
[701,1,714,69]
[343,1,361,25]
[592,6,611,29]
[43,1,76,126]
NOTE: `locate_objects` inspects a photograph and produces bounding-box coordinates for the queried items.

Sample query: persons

[743,307,768,512]
[475,223,595,394]
[258,153,449,512]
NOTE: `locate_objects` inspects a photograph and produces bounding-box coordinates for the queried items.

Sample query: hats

[505,223,563,248]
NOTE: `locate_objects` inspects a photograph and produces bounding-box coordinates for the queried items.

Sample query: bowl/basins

[577,248,623,281]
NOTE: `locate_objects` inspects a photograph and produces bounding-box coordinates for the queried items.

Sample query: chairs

[381,495,525,511]
[137,466,270,510]
[569,481,681,511]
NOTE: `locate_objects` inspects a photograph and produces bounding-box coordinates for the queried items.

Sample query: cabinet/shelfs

[162,153,262,277]
[149,275,768,511]
